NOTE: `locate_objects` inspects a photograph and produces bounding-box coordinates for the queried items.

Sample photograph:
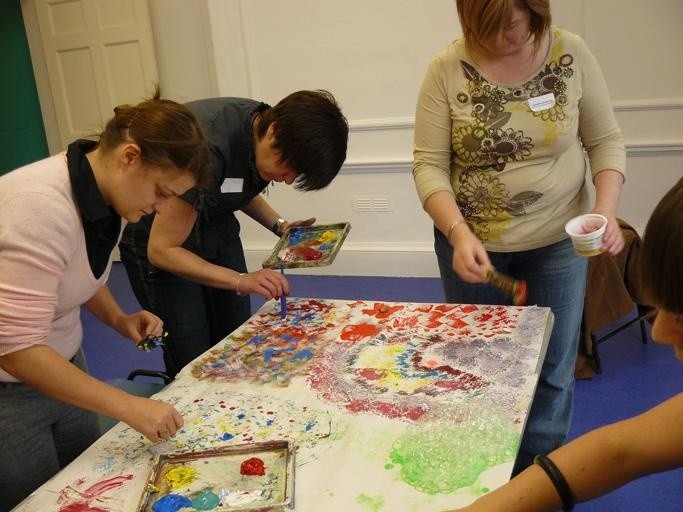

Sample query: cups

[565,214,608,260]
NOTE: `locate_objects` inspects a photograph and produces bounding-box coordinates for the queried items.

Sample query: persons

[119,90,349,383]
[449,181,683,512]
[412,1,626,479]
[0,99,206,512]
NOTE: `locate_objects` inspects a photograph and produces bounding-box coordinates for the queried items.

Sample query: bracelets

[236,273,246,297]
[447,219,467,244]
[534,455,575,512]
[273,218,287,235]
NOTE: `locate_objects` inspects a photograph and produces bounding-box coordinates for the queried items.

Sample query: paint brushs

[279,265,285,319]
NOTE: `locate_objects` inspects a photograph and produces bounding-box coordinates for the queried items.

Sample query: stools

[89,369,171,434]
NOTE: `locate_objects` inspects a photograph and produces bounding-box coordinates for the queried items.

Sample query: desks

[9,294,555,512]
